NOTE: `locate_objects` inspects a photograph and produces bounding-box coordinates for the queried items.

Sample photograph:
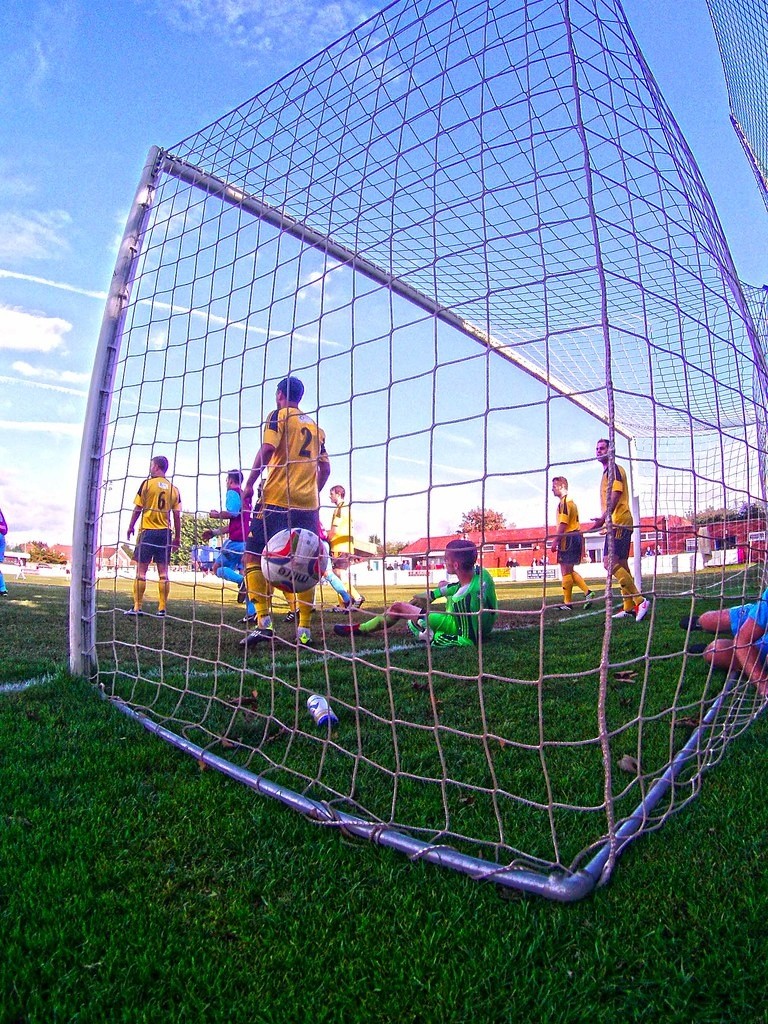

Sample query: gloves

[408,619,434,643]
[410,591,436,609]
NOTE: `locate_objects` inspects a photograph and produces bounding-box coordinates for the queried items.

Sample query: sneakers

[583,589,595,610]
[352,595,366,612]
[240,622,276,645]
[124,606,143,616]
[237,581,247,604]
[551,603,573,611]
[634,597,650,622]
[155,610,165,615]
[612,608,634,617]
[283,607,299,621]
[295,633,312,644]
[237,613,257,623]
[342,597,355,615]
[325,604,343,612]
[334,623,369,636]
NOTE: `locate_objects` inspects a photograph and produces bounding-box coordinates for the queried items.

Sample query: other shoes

[0,589,8,596]
[689,643,708,654]
[680,616,703,631]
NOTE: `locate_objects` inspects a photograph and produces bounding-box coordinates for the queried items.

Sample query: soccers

[261,526,328,593]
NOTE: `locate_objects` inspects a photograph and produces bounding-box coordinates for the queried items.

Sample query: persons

[383,559,447,571]
[201,469,300,625]
[654,544,662,555]
[66,561,72,581]
[0,507,9,597]
[531,557,539,567]
[679,586,768,696]
[36,560,40,569]
[324,485,365,615]
[123,456,182,621]
[15,558,27,580]
[333,540,498,648]
[539,554,549,566]
[645,546,652,556]
[107,559,111,570]
[240,377,331,647]
[367,563,372,571]
[313,519,355,615]
[506,557,519,567]
[589,439,650,621]
[550,476,596,611]
[583,554,591,563]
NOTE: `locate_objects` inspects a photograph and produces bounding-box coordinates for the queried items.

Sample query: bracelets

[213,529,221,536]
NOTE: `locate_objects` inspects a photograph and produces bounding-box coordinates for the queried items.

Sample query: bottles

[306,696,339,729]
